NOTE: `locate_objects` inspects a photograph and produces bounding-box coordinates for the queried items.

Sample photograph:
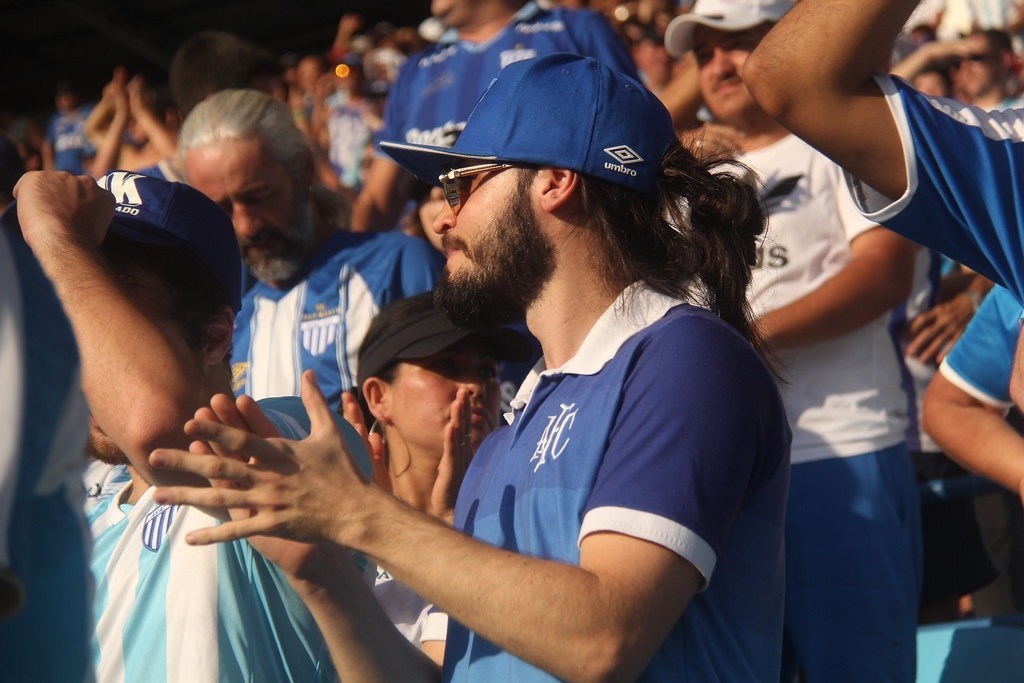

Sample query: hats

[378,54,678,197]
[357,292,534,401]
[664,0,794,59]
[86,169,243,314]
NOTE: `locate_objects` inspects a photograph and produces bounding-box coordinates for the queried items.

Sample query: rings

[457,442,466,448]
[694,139,704,149]
[464,433,473,438]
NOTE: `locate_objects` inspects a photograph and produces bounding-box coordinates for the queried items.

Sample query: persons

[904,247,1019,626]
[335,294,533,683]
[147,41,790,683]
[164,81,462,683]
[1,161,374,680]
[658,1,922,680]
[925,273,1024,499]
[742,0,1022,435]
[1,0,1022,277]
